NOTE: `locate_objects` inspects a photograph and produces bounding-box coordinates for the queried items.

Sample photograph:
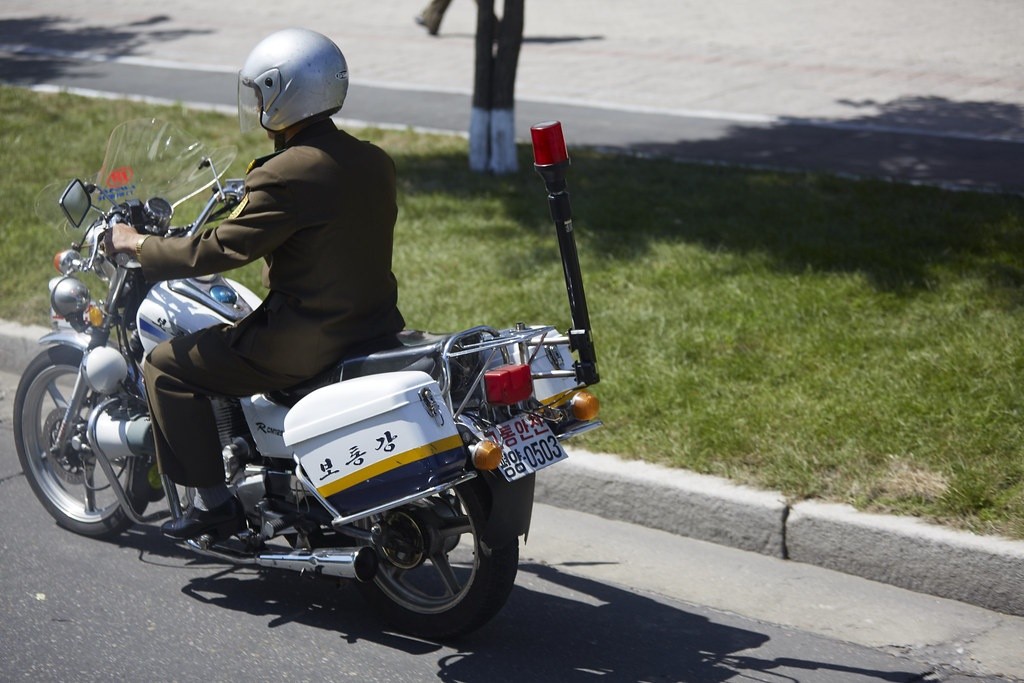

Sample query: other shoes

[414,13,441,35]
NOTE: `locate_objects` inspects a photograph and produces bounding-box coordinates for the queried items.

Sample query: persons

[112,31,402,540]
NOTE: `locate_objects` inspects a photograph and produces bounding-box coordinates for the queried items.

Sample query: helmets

[240,28,348,134]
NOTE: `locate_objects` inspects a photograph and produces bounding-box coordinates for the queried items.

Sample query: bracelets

[135,234,152,263]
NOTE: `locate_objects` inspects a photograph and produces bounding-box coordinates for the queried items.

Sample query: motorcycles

[6,107,610,644]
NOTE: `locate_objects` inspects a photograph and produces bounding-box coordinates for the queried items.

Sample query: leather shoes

[160,495,246,540]
